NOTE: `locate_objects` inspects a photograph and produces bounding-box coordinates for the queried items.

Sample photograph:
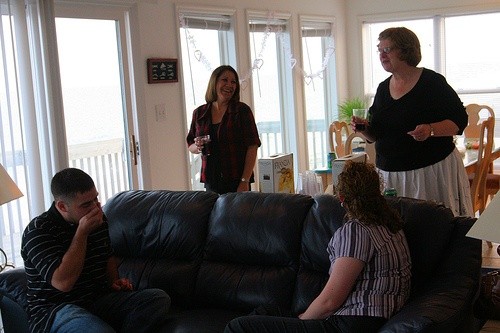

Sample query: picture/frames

[147,58,179,84]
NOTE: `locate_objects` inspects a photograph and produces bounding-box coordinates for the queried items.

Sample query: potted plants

[339,97,369,125]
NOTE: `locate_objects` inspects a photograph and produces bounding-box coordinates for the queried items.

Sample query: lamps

[0,165,23,272]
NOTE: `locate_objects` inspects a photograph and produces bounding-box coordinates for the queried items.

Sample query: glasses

[378,47,396,52]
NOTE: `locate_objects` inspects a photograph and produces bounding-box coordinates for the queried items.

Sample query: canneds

[327,153,336,169]
[384,188,397,197]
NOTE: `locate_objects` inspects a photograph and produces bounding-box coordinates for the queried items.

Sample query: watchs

[239,177,250,183]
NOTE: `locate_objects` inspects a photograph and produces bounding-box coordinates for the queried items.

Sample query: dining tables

[453,137,500,174]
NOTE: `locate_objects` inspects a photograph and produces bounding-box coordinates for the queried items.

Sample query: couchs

[0,189,482,333]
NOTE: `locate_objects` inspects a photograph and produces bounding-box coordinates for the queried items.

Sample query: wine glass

[197,135,211,157]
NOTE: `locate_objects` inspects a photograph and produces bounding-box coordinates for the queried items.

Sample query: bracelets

[351,26,474,218]
[428,124,435,137]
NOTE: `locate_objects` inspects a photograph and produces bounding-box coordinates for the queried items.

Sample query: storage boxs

[332,152,366,186]
[257,152,295,193]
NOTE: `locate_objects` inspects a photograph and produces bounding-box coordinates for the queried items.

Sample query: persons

[186,65,262,195]
[224,159,412,333]
[21,168,172,333]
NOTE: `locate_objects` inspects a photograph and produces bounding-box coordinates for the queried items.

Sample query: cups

[353,109,368,131]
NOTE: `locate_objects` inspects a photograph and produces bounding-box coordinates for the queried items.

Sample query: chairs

[328,121,352,157]
[465,105,494,200]
[469,117,495,216]
